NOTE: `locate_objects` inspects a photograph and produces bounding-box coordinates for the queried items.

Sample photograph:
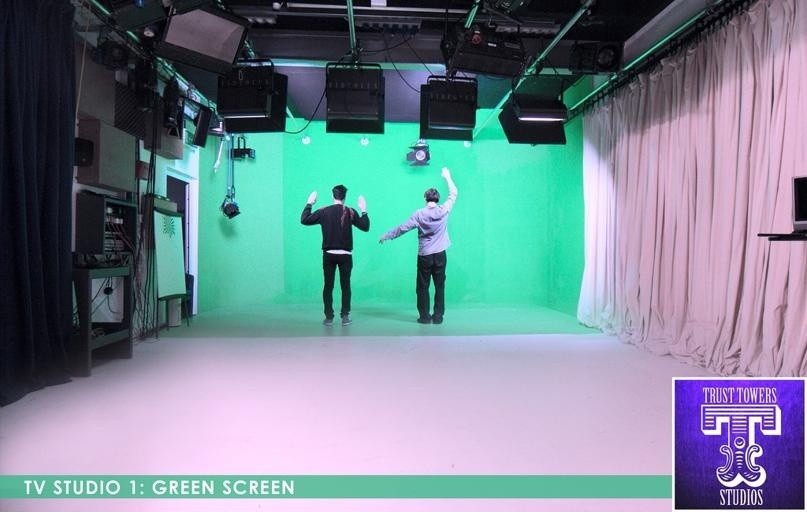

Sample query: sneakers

[342,314,352,326]
[323,316,333,326]
[417,314,443,324]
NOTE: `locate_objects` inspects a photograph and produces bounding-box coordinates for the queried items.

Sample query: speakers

[193,108,212,147]
[157,2,250,75]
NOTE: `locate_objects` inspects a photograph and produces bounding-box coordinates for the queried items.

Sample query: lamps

[498,61,567,144]
[213,56,288,133]
[406,138,430,167]
[415,66,477,141]
[221,134,240,218]
[323,47,385,136]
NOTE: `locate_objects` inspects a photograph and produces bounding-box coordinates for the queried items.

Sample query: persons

[299,183,371,326]
[377,167,458,325]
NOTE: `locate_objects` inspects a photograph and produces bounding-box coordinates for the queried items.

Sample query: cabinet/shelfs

[69,268,134,378]
